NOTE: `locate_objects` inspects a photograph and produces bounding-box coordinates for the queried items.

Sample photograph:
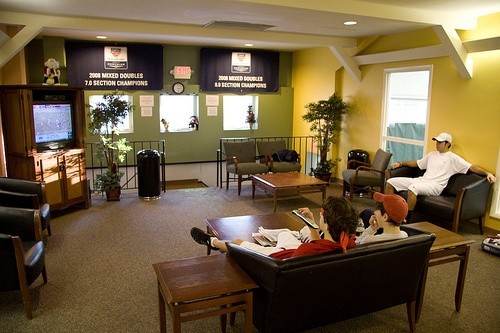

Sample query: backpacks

[481,237,500,257]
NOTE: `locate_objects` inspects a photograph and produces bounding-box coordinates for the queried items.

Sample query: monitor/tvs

[33,101,76,151]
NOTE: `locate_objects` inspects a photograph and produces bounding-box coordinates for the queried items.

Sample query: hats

[373,192,409,223]
[432,133,452,145]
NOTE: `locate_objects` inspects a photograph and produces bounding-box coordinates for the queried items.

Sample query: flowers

[246,111,257,124]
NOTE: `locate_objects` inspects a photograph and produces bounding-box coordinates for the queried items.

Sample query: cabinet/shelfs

[0,88,89,212]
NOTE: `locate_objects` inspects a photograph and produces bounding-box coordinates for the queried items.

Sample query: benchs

[225,224,436,333]
[384,165,496,235]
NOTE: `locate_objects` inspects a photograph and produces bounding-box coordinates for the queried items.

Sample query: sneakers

[190,227,220,251]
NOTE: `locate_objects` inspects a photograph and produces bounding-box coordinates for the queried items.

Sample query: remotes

[293,210,319,229]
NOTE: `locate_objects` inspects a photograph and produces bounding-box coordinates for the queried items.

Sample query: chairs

[341,148,393,202]
[222,140,302,196]
[0,176,52,319]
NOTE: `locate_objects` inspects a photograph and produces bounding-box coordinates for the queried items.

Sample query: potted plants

[301,91,351,186]
[83,89,134,201]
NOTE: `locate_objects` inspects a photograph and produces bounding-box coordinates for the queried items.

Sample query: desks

[152,254,261,333]
[406,221,476,323]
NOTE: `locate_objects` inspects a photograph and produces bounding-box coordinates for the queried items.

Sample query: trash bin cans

[345,149,370,198]
[136,149,161,200]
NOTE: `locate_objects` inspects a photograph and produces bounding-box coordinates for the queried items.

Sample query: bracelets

[399,162,402,167]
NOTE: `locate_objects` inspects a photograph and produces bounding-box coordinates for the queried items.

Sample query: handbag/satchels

[272,150,298,162]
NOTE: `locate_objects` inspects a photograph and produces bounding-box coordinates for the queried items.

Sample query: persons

[384,133,496,223]
[190,193,409,261]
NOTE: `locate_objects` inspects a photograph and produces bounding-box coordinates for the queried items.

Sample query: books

[253,227,300,246]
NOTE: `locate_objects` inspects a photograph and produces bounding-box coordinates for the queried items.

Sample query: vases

[250,122,254,130]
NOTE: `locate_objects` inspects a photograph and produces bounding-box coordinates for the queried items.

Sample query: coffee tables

[204,208,320,254]
[249,171,329,213]
